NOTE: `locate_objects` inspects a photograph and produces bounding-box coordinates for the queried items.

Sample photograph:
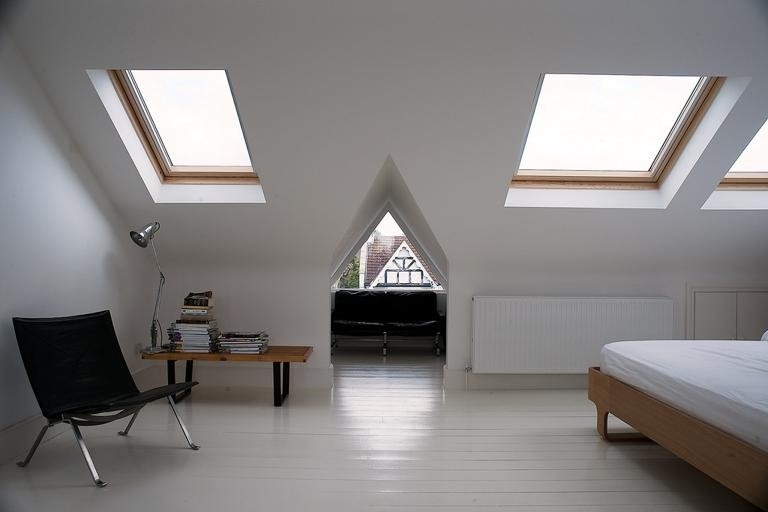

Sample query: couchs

[330,290,445,357]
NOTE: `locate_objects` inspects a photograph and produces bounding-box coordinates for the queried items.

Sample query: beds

[589,340,768,512]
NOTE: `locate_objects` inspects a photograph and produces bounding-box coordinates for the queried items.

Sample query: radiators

[465,296,675,376]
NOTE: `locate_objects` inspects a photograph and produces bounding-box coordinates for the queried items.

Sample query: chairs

[13,307,199,487]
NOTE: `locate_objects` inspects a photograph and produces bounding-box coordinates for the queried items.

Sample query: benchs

[141,346,312,406]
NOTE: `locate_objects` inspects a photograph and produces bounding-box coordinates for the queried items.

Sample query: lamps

[129,221,169,352]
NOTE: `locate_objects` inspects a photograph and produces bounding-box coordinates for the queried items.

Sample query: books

[166,290,270,357]
